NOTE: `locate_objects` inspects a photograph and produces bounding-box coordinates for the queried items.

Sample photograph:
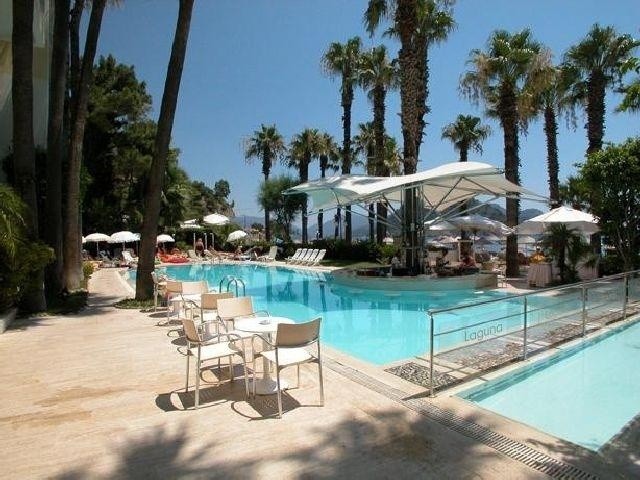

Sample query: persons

[157,238,242,260]
[84,248,139,261]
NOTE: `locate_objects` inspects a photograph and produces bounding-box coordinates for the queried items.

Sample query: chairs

[81,247,139,266]
[157,242,330,267]
[146,270,327,420]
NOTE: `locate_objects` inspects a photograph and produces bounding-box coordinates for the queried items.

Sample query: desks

[425,248,601,287]
